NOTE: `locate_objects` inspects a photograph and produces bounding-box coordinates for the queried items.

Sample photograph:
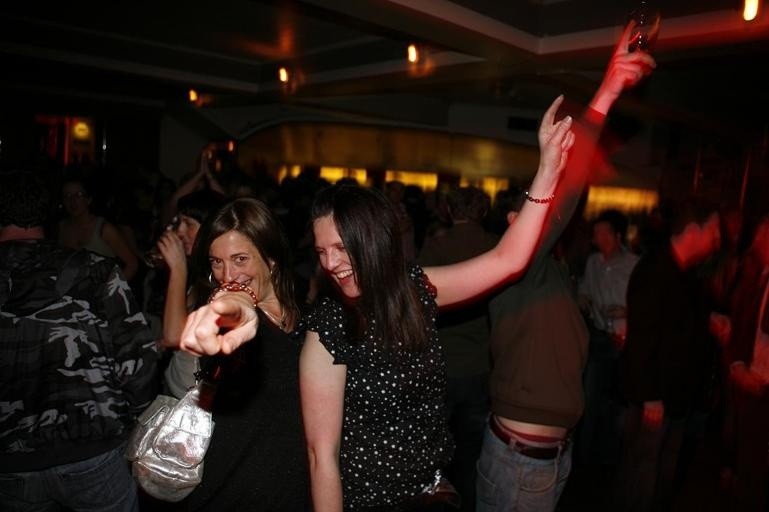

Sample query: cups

[142,245,166,269]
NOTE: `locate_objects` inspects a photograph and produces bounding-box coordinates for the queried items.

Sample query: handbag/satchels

[122,378,214,501]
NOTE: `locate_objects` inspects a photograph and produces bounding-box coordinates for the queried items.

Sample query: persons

[297,95,575,511]
[244,169,451,300]
[1,145,255,512]
[178,199,309,510]
[406,17,767,511]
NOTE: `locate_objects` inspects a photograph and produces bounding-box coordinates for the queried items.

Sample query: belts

[486,414,573,462]
